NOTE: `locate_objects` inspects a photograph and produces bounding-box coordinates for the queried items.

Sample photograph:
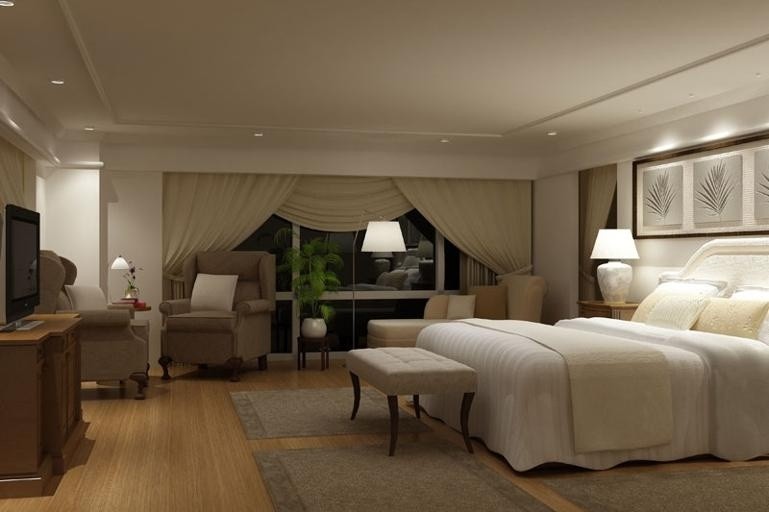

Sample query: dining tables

[275,226,345,339]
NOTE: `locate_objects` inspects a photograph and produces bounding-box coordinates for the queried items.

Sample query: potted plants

[633,130,769,239]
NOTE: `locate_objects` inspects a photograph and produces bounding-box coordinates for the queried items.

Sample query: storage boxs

[346,347,478,458]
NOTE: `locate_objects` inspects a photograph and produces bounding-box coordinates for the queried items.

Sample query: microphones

[296,336,329,371]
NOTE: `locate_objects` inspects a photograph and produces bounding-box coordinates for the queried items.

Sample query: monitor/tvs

[107,299,145,308]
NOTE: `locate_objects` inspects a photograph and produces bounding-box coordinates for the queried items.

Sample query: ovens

[414,239,769,473]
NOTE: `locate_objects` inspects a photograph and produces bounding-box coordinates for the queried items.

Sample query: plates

[0,313,96,499]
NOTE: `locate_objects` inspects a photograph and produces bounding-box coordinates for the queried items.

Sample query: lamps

[367,274,547,349]
[158,250,275,383]
[36,250,150,401]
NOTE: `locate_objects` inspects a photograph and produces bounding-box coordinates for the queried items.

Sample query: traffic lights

[191,272,239,312]
[692,297,769,341]
[63,284,108,310]
[629,281,718,333]
[468,285,507,319]
[657,277,732,297]
[732,284,769,345]
[423,294,447,320]
[448,295,476,319]
[377,272,407,288]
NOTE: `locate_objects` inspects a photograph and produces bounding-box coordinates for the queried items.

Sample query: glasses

[110,257,138,299]
[589,228,640,303]
[352,210,407,350]
[371,252,393,279]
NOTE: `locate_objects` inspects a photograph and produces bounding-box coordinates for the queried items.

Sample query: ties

[0,203,45,331]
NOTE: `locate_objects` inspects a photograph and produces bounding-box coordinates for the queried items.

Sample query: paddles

[228,387,434,440]
[540,465,769,512]
[253,439,557,512]
